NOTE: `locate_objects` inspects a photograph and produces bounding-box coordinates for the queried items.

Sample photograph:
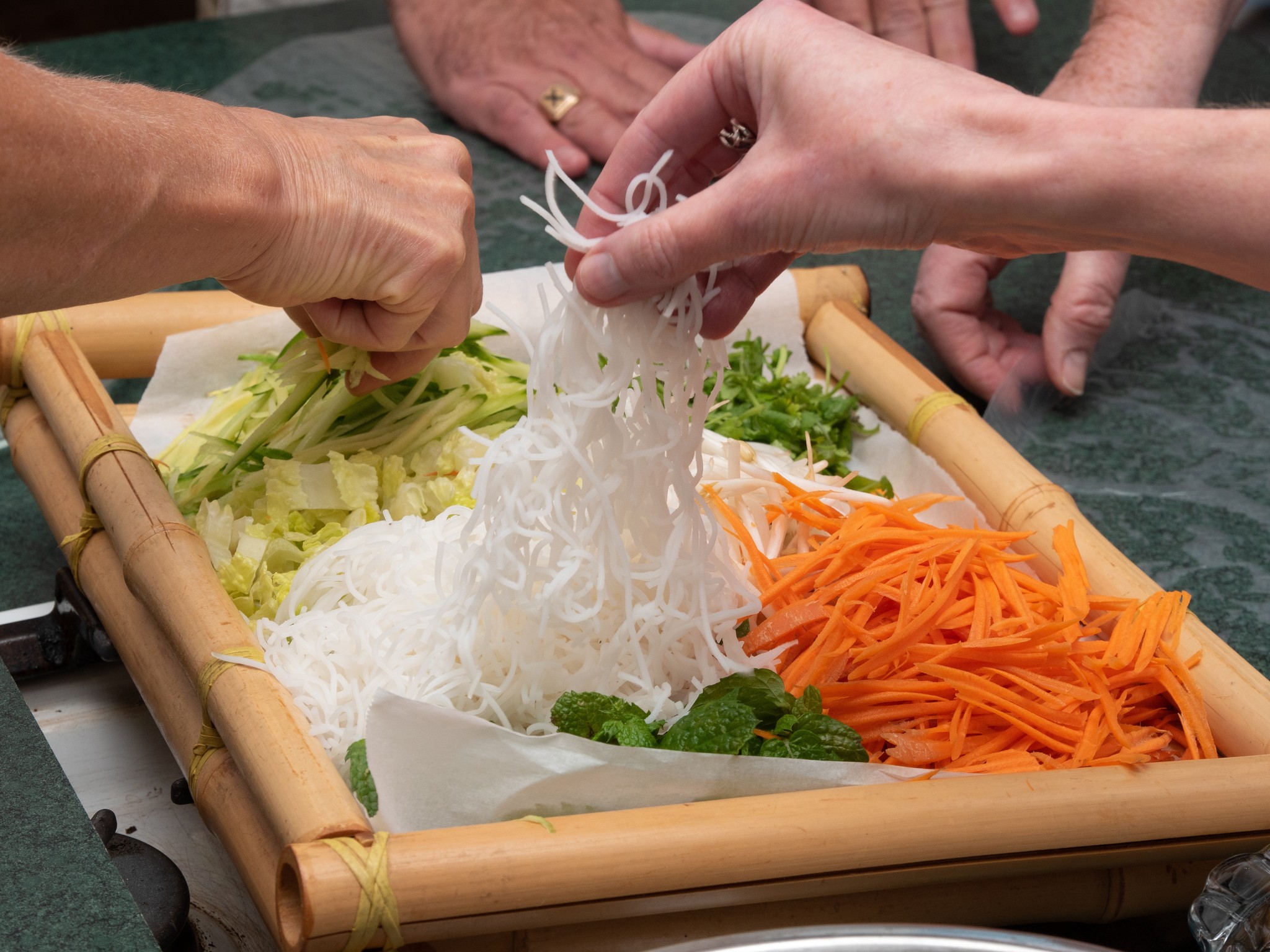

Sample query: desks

[1,1,1270,952]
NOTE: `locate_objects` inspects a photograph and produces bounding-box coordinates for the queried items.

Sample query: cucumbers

[155,309,536,505]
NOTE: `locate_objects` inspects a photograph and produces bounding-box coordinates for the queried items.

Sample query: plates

[649,918,1114,952]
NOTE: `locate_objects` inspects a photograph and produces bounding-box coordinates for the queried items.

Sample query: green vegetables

[593,330,895,502]
[346,669,873,819]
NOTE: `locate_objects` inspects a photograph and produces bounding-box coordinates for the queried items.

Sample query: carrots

[697,470,1219,777]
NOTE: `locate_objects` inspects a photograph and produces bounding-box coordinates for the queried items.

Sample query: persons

[387,1,707,172]
[804,1,1041,79]
[909,2,1261,414]
[564,2,1268,344]
[1,48,485,398]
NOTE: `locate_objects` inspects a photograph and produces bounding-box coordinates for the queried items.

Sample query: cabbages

[168,374,489,617]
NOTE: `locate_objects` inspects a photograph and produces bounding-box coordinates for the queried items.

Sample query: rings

[540,78,586,126]
